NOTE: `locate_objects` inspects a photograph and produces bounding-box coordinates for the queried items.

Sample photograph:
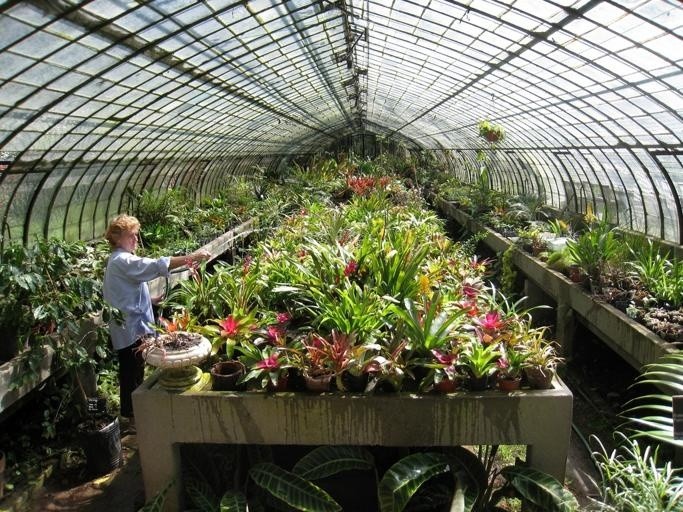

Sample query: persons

[101,215,210,434]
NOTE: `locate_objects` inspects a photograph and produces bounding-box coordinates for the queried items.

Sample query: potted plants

[0,152,683,510]
